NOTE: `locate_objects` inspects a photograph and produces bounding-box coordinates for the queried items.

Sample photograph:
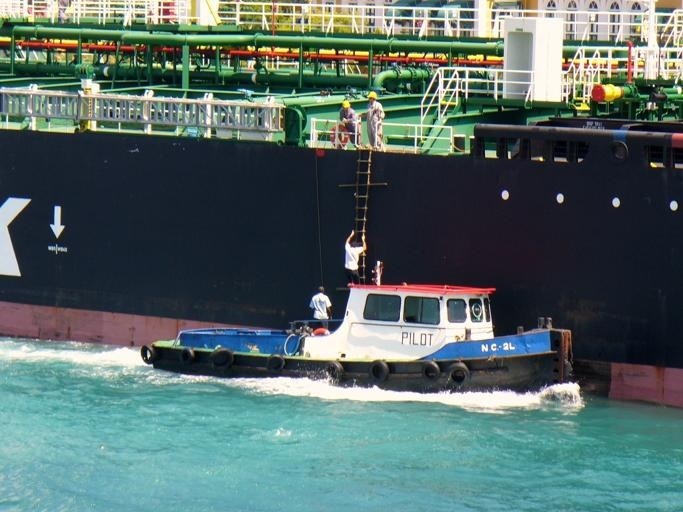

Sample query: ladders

[353,148,373,284]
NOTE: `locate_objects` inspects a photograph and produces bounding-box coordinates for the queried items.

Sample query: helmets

[343,100,350,108]
[367,91,377,99]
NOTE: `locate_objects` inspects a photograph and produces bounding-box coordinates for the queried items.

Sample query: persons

[56,0,70,24]
[354,91,386,149]
[338,100,362,149]
[305,286,333,331]
[343,229,367,285]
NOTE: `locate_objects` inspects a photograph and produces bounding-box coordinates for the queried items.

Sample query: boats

[142,280,574,401]
[0,2,683,407]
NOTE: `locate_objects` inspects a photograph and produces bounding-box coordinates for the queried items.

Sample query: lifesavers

[310,328,331,336]
[330,124,349,149]
